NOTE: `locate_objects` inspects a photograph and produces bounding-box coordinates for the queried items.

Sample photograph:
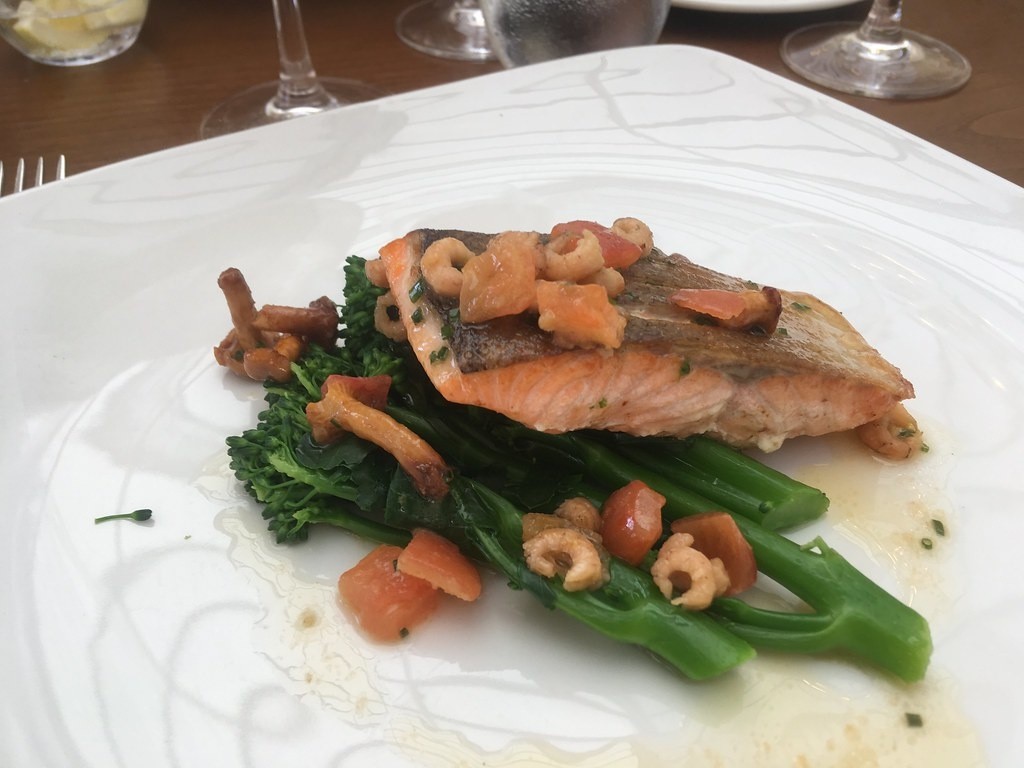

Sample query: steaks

[378,228,917,452]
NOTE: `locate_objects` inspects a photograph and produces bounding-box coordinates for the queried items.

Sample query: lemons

[10,0,146,52]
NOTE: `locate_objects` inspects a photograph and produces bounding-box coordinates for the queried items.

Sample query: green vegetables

[224,254,933,684]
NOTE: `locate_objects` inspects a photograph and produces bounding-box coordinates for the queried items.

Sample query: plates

[0,42,1024,768]
[664,0,867,14]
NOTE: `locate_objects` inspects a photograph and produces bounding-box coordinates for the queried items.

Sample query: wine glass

[197,0,390,141]
[394,0,498,62]
[777,0,971,104]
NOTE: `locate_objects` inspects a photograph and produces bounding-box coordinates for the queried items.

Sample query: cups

[0,0,149,68]
[476,1,671,69]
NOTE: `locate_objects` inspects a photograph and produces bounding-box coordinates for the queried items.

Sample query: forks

[0,153,67,198]
